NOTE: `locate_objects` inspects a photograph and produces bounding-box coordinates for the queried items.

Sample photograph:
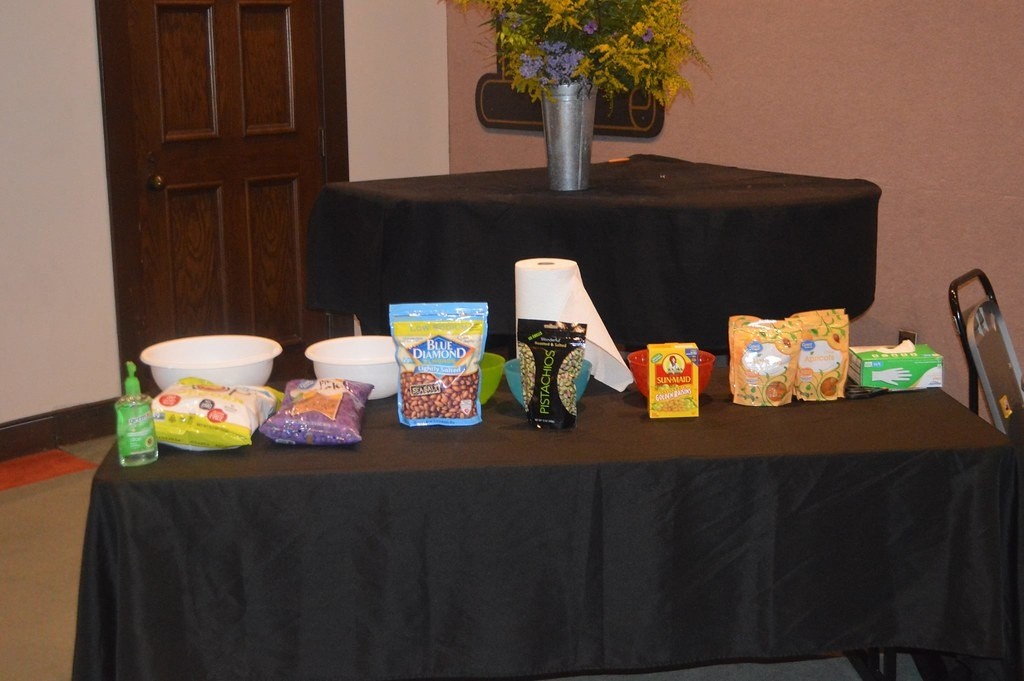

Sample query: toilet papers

[515,258,634,392]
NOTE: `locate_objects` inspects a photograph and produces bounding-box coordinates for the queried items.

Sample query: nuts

[400,371,478,420]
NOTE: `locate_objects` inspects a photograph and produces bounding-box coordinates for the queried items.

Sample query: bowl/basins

[475,351,593,413]
[627,350,715,403]
[139,333,284,392]
[304,335,398,400]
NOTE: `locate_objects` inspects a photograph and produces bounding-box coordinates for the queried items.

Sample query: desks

[71,354,1024,681]
[302,150,882,346]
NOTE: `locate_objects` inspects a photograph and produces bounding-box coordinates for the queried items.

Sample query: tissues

[848,339,944,391]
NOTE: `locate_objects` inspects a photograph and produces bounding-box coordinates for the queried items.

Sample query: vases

[543,76,597,192]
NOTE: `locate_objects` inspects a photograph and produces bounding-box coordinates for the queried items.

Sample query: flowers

[444,0,713,114]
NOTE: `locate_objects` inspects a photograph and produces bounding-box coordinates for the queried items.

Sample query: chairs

[947,269,1000,417]
